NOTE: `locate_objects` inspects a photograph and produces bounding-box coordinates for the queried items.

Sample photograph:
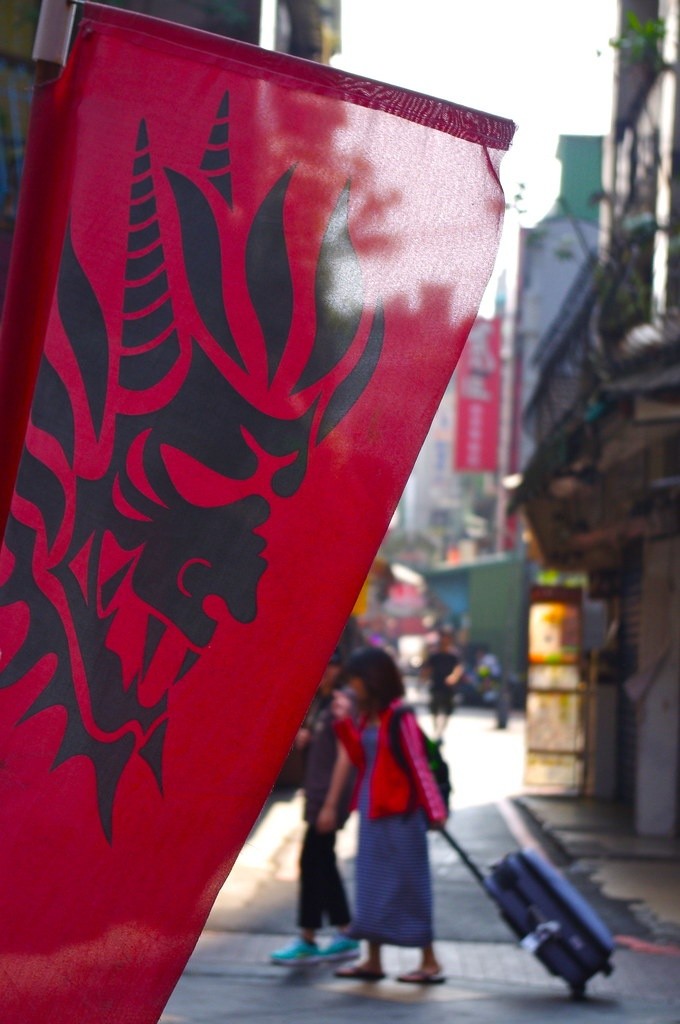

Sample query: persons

[267,651,364,965]
[472,641,510,730]
[420,624,464,743]
[332,645,448,984]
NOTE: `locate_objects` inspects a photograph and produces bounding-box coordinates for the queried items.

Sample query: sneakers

[320,935,360,958]
[271,936,321,965]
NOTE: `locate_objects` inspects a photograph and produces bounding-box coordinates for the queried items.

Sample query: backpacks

[388,706,452,826]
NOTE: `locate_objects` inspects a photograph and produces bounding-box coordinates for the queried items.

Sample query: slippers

[335,964,386,979]
[398,971,446,984]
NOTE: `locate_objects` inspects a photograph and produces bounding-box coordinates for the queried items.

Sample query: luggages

[440,826,614,998]
